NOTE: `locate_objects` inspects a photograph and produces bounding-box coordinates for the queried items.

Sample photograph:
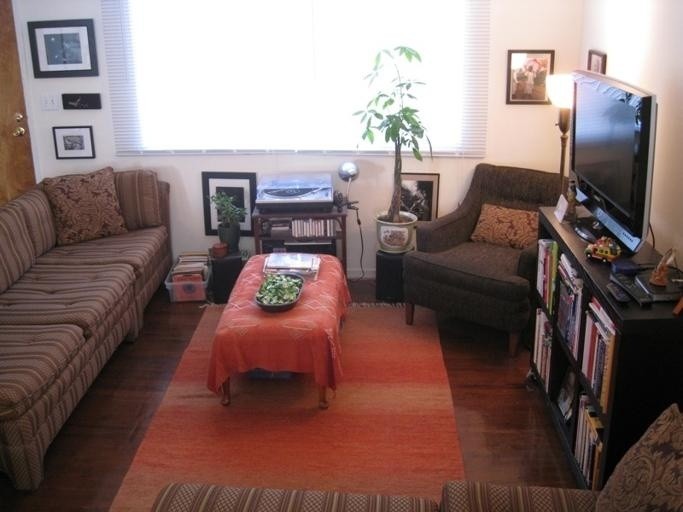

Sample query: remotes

[605,279,630,303]
[610,272,652,309]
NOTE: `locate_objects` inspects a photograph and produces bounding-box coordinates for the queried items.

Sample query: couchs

[144,477,608,511]
[397,159,573,346]
[0,166,177,498]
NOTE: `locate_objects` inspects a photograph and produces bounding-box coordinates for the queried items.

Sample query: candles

[353,45,429,257]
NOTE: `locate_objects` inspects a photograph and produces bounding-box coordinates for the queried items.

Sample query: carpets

[107,301,474,512]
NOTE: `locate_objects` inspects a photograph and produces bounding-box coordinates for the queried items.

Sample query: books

[261,250,321,281]
[532,236,621,488]
[268,217,337,238]
[170,249,210,302]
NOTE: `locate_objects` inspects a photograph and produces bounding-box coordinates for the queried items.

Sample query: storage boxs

[164,252,213,302]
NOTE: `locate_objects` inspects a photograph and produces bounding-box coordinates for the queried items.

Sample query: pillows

[43,167,129,245]
[592,399,682,510]
[469,202,539,253]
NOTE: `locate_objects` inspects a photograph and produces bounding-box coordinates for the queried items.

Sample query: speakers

[376,250,410,305]
[210,252,242,305]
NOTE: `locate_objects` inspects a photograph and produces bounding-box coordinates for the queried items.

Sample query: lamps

[544,69,578,174]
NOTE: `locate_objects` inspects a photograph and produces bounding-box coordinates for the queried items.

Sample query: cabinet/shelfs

[523,201,683,494]
[248,191,350,286]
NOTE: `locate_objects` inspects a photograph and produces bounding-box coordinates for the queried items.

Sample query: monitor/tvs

[568,68,658,259]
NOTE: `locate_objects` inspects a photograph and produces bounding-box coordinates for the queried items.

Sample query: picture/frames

[201,170,257,238]
[587,48,608,75]
[399,172,441,229]
[52,124,96,160]
[27,19,100,80]
[504,47,556,106]
